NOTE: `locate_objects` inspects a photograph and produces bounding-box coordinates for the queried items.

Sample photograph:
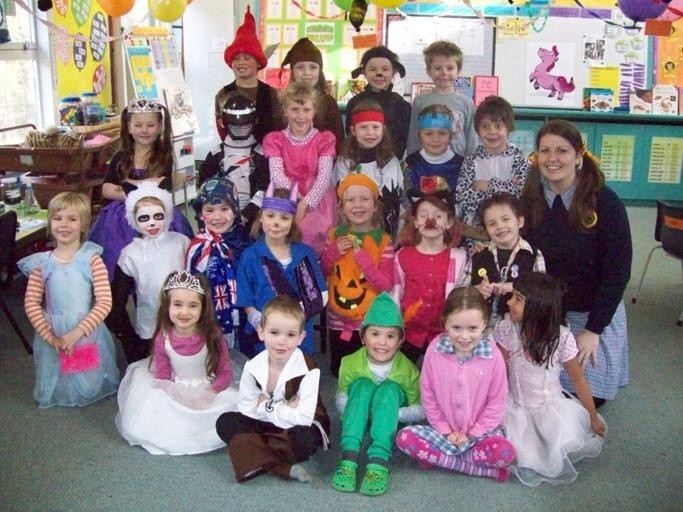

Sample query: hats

[277,36,324,90]
[349,45,406,80]
[221,4,268,72]
[358,290,405,341]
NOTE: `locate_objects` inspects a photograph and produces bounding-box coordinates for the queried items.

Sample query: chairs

[0,208,33,360]
[627,198,682,328]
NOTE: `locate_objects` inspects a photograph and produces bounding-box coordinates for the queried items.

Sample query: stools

[306,301,331,352]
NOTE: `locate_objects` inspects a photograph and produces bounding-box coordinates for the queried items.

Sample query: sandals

[359,462,391,497]
[329,458,359,494]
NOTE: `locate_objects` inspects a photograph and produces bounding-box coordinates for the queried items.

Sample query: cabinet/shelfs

[166,129,197,207]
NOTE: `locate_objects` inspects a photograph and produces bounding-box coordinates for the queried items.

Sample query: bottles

[23,182,41,215]
[0,177,22,205]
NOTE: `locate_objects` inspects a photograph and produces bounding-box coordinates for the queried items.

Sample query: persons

[474,121,633,407]
[85,100,194,287]
[214,39,288,145]
[236,190,330,373]
[389,197,467,364]
[215,295,332,484]
[454,194,547,333]
[329,291,424,494]
[18,190,127,409]
[320,174,396,378]
[281,39,348,151]
[185,175,254,351]
[112,184,194,363]
[456,94,530,248]
[344,45,413,161]
[396,286,516,482]
[403,40,479,158]
[113,269,249,457]
[400,103,467,212]
[194,94,271,229]
[491,271,607,486]
[263,82,340,260]
[331,98,405,221]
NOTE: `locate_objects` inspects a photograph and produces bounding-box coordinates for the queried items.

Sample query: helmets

[220,94,258,141]
[191,177,243,232]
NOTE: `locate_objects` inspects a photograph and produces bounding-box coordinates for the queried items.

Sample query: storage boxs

[0,130,122,175]
[29,177,106,210]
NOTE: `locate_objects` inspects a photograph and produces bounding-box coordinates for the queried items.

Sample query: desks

[0,200,51,250]
